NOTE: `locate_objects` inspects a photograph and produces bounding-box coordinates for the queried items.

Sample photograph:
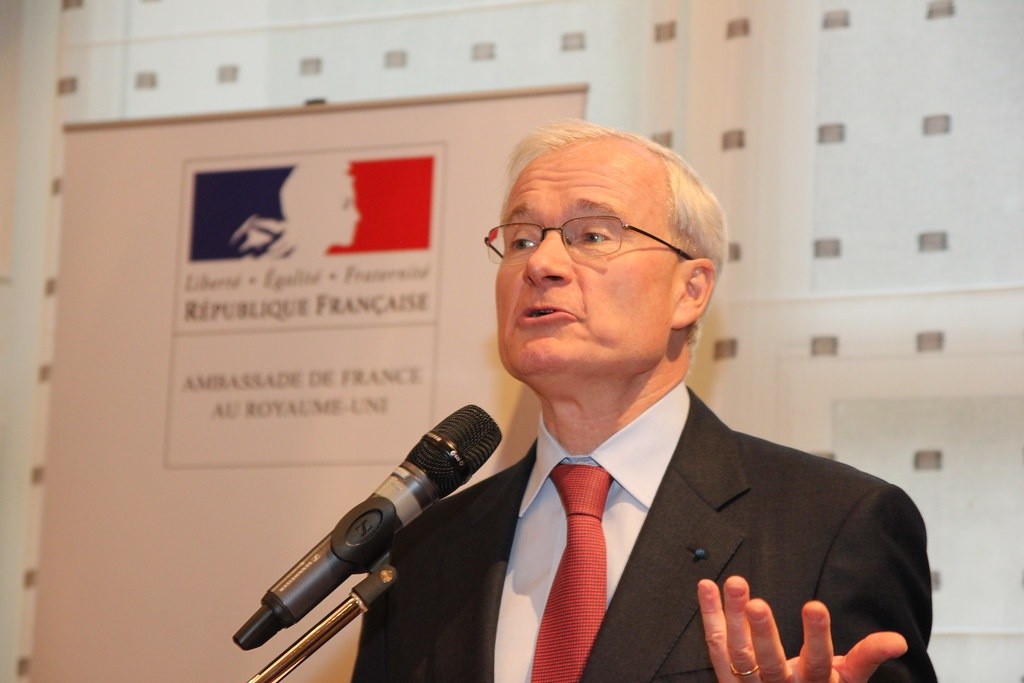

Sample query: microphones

[233,405,501,651]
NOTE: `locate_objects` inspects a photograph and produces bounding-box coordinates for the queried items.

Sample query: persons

[352,117,940,683]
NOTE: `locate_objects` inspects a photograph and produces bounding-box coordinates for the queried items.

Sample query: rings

[730,663,758,675]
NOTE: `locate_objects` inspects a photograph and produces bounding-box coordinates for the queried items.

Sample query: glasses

[484,215,693,265]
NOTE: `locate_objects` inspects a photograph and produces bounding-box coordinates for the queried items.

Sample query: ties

[530,464,613,683]
